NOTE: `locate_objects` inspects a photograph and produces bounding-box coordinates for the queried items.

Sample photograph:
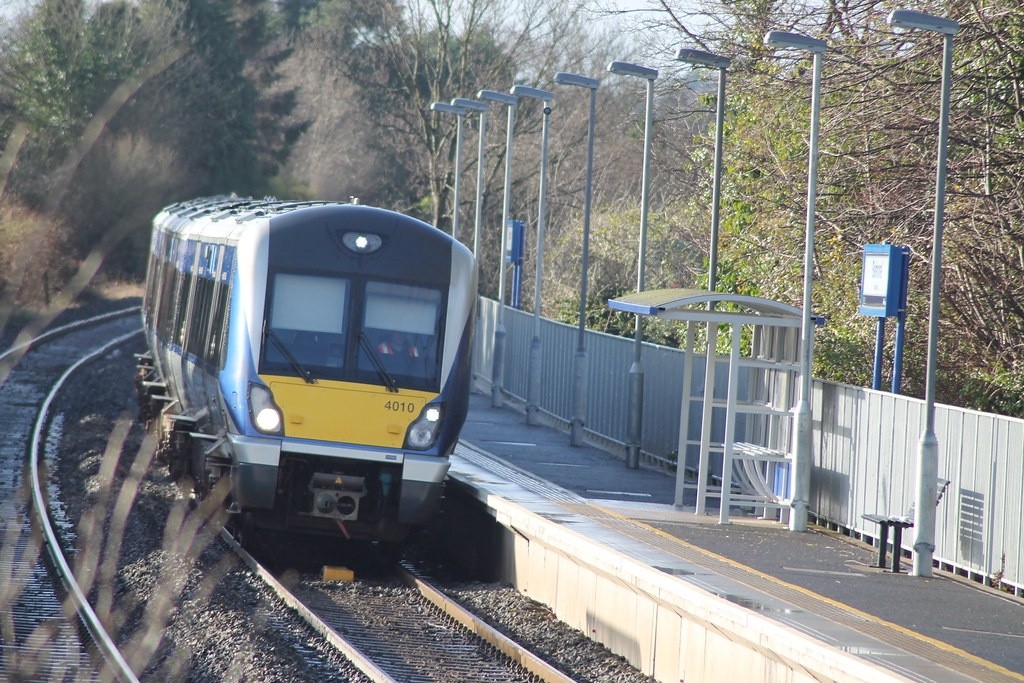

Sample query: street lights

[449,96,488,262]
[763,29,827,530]
[477,89,517,410]
[429,100,471,242]
[606,60,658,470]
[886,6,959,575]
[554,72,600,446]
[510,84,558,427]
[671,48,731,311]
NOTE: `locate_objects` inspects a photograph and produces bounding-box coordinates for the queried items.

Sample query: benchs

[862,477,949,573]
[721,439,792,522]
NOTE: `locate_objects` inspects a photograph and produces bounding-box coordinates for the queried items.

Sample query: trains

[134,196,478,561]
